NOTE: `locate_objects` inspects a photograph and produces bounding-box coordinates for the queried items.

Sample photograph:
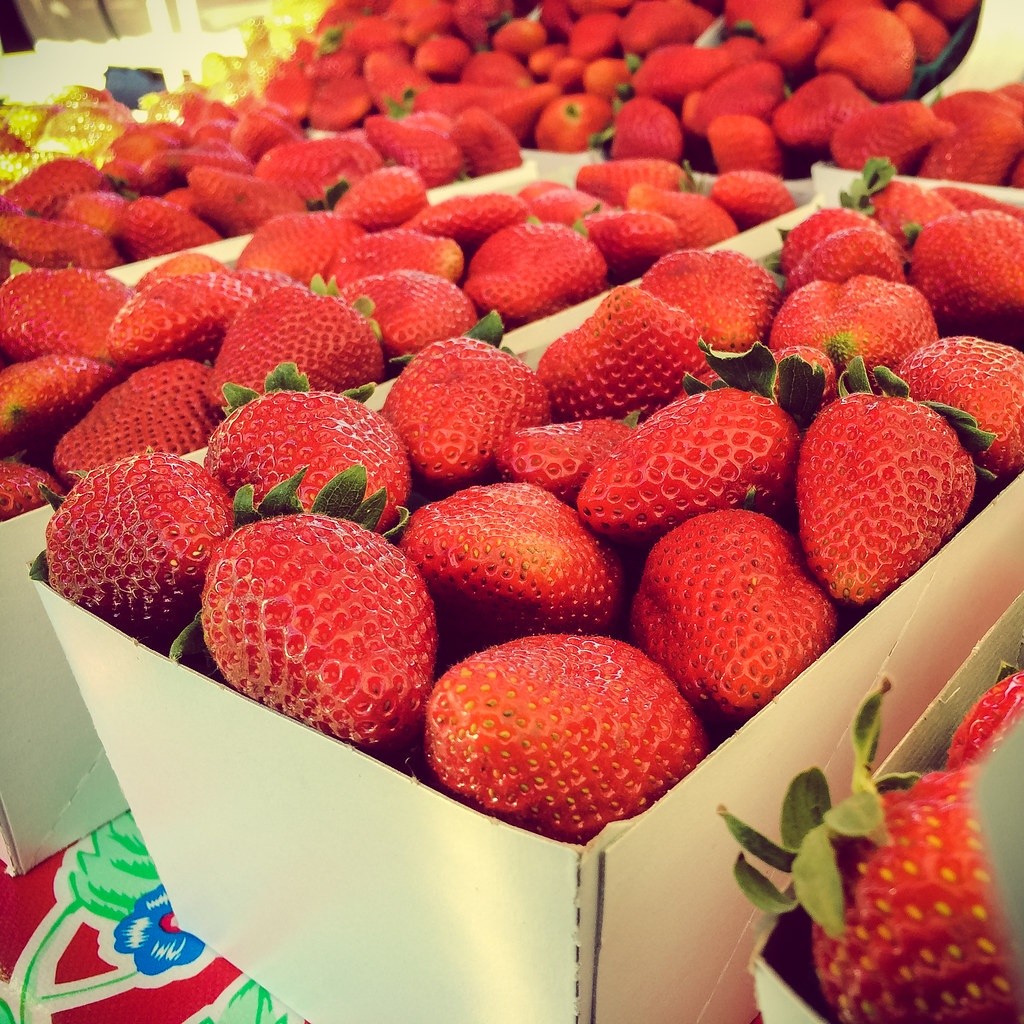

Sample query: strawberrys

[0,0,1024,1024]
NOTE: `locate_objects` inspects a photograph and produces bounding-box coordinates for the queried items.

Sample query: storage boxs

[0,0,1023,1024]
